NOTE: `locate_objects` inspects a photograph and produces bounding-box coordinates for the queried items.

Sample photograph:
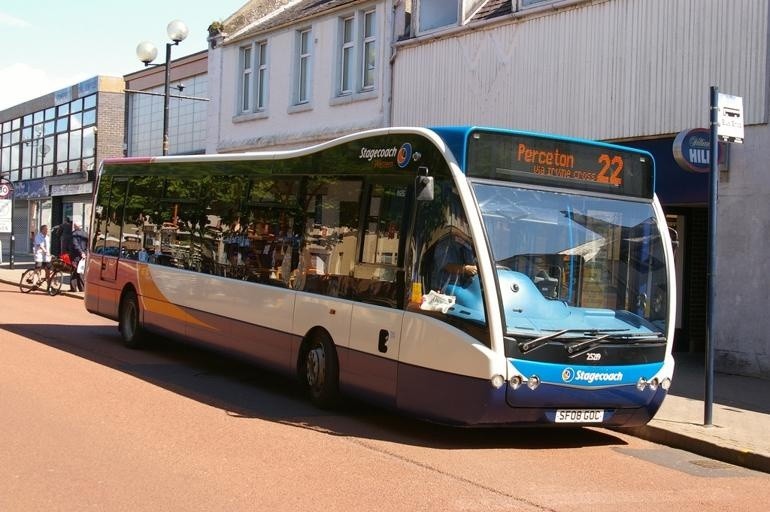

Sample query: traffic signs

[0,184,10,199]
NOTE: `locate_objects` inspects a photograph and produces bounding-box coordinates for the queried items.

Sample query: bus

[82,125,677,432]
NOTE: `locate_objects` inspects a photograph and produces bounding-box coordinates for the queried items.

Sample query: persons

[68,223,89,293]
[430,212,502,297]
[25,223,59,293]
[53,217,72,258]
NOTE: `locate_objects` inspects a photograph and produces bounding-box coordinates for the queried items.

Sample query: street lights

[120,18,210,156]
[24,131,50,178]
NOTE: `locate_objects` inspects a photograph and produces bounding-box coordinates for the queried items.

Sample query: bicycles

[19,256,75,296]
